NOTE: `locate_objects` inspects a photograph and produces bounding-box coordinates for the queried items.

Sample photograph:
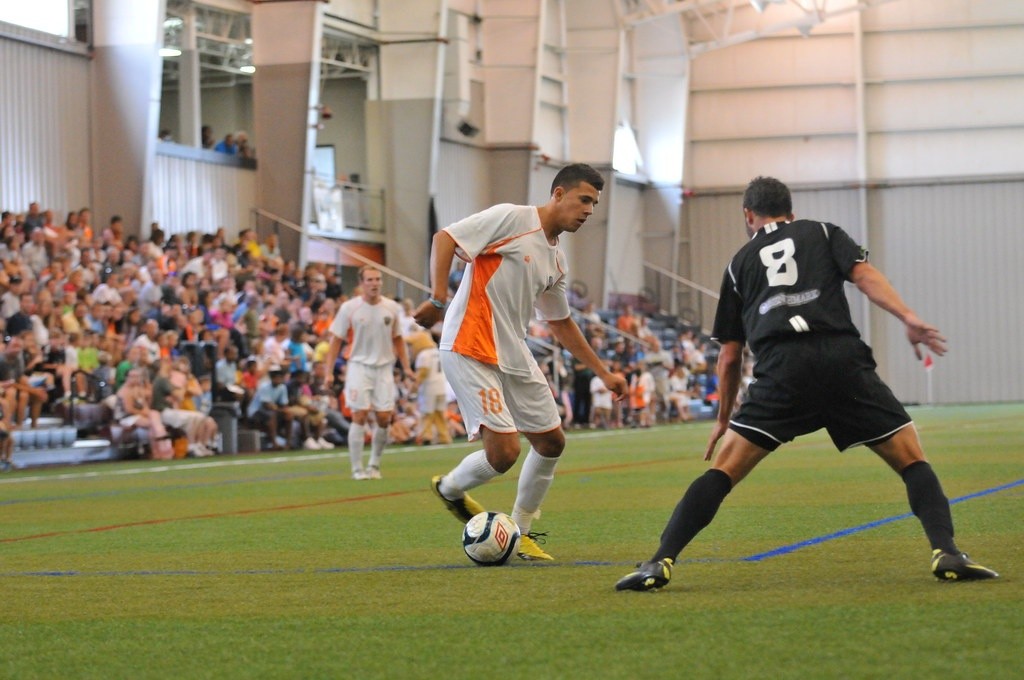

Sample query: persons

[202,126,215,149]
[615,177,1000,592]
[524,299,755,429]
[412,163,630,561]
[0,202,467,470]
[323,265,416,480]
[161,130,172,140]
[214,134,237,154]
[449,261,465,291]
[235,132,251,157]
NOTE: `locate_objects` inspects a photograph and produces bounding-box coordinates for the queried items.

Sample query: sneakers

[186,443,204,457]
[517,530,555,561]
[316,436,334,449]
[616,556,674,592]
[431,474,486,524]
[195,442,214,457]
[302,437,321,450]
[931,548,1000,582]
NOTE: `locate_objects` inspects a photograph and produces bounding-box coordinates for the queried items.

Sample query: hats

[268,365,286,376]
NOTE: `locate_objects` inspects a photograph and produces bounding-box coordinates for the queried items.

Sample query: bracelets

[429,298,445,308]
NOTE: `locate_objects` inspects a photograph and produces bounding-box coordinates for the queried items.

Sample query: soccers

[461,510,522,566]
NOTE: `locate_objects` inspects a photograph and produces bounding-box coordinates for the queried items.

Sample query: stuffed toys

[407,333,453,445]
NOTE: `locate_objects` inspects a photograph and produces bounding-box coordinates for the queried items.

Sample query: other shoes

[365,468,382,480]
[153,448,175,460]
[564,416,657,430]
[351,470,370,480]
